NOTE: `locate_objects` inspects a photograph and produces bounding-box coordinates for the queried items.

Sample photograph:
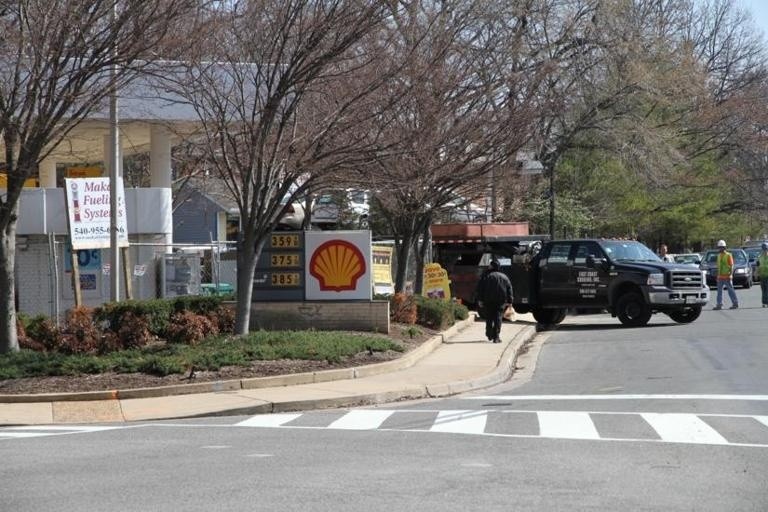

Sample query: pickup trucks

[449,238,709,328]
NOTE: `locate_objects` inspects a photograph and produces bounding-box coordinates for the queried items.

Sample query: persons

[713,239,738,310]
[657,244,674,262]
[759,243,768,308]
[477,259,514,343]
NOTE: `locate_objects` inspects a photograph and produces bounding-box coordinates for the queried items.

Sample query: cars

[666,240,768,288]
[271,187,503,230]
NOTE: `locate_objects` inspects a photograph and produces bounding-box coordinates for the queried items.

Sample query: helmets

[717,239,727,247]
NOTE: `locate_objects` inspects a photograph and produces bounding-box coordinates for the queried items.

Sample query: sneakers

[488,336,503,344]
[712,304,738,310]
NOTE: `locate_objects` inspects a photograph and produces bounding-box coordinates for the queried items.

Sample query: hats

[762,243,768,250]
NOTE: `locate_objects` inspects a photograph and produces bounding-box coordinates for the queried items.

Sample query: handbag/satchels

[503,305,518,322]
